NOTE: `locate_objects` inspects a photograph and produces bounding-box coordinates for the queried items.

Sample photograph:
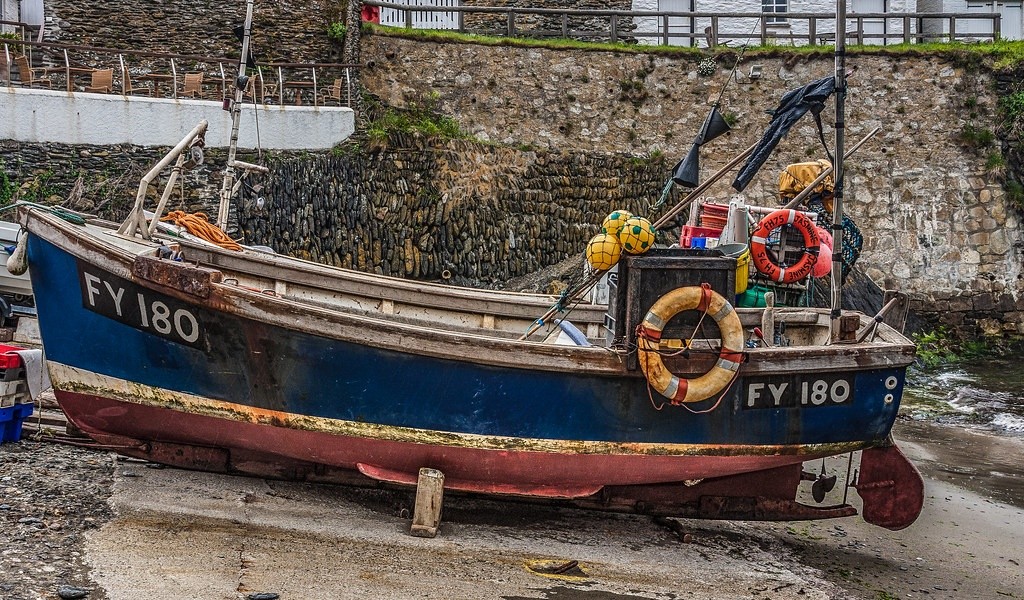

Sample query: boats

[0,344,40,445]
[16,117,926,538]
[0,220,32,297]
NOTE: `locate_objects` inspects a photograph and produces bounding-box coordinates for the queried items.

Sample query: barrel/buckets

[706,237,719,248]
[715,244,750,294]
[739,286,773,307]
[692,237,705,249]
[701,203,729,218]
[700,215,728,230]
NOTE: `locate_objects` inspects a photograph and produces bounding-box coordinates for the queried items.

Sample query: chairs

[0,51,342,107]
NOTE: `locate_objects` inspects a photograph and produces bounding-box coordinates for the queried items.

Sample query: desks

[203,79,233,101]
[56,67,98,92]
[284,82,314,106]
[137,74,174,98]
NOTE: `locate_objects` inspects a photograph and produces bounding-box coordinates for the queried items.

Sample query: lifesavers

[750,208,821,283]
[638,286,745,403]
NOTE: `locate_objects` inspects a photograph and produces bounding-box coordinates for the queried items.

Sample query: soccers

[602,210,634,247]
[620,216,656,255]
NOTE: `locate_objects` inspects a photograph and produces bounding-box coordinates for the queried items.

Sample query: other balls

[586,233,620,270]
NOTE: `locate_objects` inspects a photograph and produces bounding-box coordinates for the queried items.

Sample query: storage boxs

[583,203,738,339]
[0,344,33,445]
